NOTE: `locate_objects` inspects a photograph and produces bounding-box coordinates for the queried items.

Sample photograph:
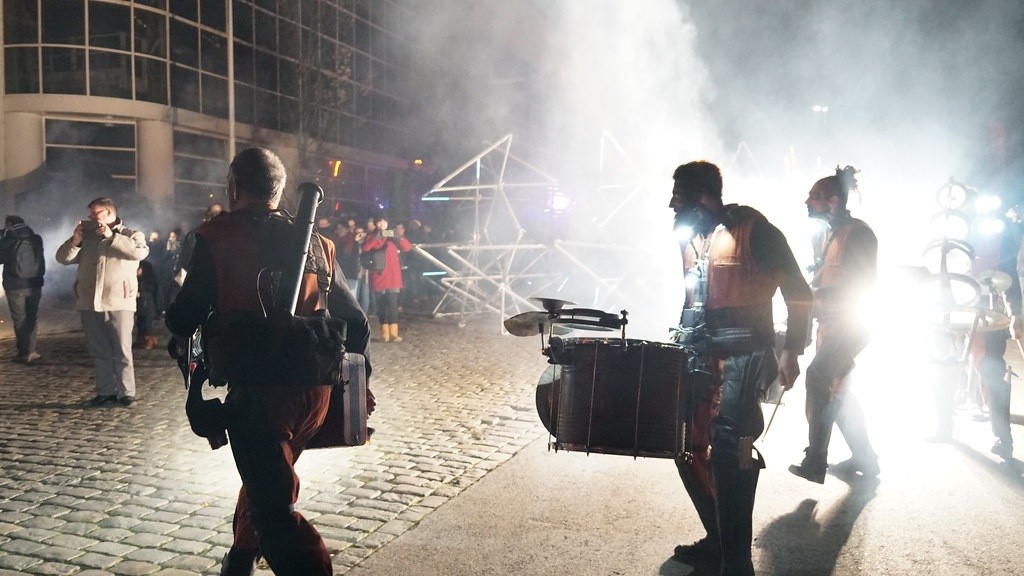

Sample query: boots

[379,323,390,343]
[389,322,403,342]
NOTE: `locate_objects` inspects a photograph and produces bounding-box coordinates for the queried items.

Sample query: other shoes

[120,396,138,408]
[990,440,1013,462]
[143,335,157,350]
[82,395,117,406]
[787,453,829,484]
[832,452,880,477]
[687,557,756,576]
[675,531,722,565]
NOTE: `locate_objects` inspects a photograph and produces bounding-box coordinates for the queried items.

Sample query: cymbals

[173,334,194,390]
[503,296,621,337]
[534,362,567,438]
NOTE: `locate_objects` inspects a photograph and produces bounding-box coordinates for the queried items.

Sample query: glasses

[87,207,108,220]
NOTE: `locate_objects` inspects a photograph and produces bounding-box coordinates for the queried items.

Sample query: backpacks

[11,229,45,280]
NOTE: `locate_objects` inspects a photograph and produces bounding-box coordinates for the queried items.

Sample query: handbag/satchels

[359,240,388,270]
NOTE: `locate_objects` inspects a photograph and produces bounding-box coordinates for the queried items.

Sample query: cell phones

[81,220,99,232]
[361,233,367,237]
[382,230,394,237]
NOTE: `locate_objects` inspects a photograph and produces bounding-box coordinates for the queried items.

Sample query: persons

[55,197,150,409]
[668,160,1023,572]
[0,213,45,365]
[137,202,478,351]
[163,145,369,575]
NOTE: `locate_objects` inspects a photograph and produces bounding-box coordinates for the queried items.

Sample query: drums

[305,352,368,451]
[763,329,787,405]
[554,336,699,464]
